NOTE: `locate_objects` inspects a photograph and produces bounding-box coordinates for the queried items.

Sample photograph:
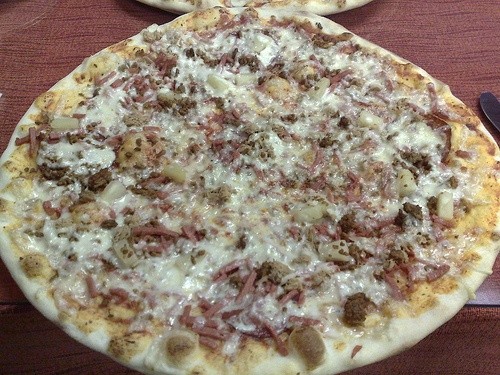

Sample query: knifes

[480,91,500,133]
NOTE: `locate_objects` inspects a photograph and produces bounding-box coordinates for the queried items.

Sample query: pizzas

[0,0,500,375]
